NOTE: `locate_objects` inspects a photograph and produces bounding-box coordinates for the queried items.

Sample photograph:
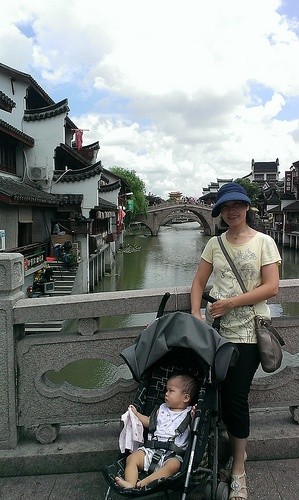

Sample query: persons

[115,374,198,489]
[190,183,282,500]
[54,241,61,260]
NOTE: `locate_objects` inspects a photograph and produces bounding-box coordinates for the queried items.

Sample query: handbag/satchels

[254,315,285,373]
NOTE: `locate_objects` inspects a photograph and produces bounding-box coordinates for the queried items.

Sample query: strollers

[104,292,232,499]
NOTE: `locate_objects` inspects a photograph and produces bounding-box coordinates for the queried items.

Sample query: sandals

[217,450,247,482]
[229,470,247,500]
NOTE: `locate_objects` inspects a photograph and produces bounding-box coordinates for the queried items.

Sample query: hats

[211,183,251,218]
